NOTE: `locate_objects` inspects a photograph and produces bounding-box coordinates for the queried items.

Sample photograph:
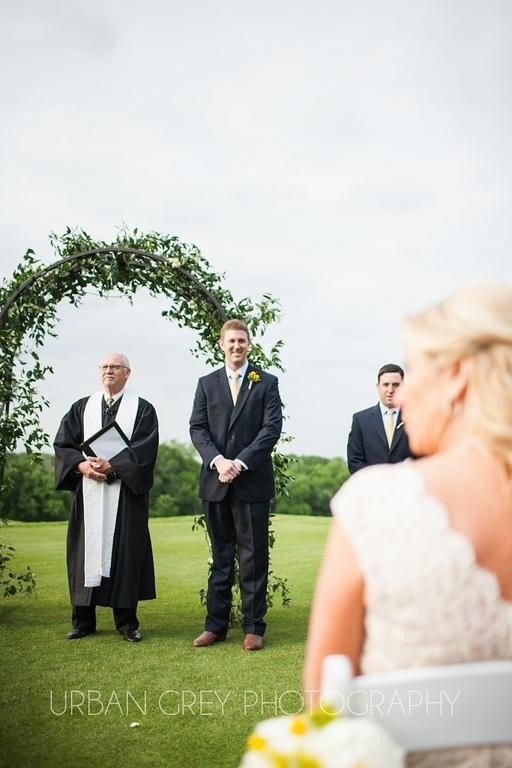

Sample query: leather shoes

[193,631,226,646]
[66,629,89,639]
[123,630,142,642]
[243,633,263,650]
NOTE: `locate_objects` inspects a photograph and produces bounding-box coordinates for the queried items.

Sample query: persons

[189,319,284,651]
[347,364,416,476]
[303,275,510,767]
[52,354,160,643]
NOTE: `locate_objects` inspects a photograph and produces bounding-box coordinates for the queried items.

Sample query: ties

[106,397,114,410]
[229,372,241,406]
[385,409,394,449]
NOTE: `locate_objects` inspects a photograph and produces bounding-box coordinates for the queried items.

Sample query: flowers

[247,371,262,389]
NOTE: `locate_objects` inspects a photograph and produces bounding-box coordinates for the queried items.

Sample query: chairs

[320,655,512,768]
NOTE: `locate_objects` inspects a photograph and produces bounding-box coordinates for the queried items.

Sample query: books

[78,420,131,485]
